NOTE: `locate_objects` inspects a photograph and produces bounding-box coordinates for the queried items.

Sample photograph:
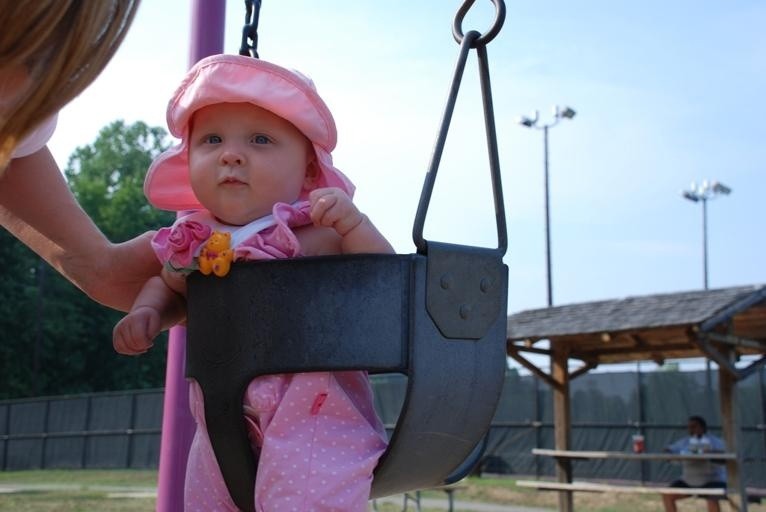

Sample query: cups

[632,435,645,454]
[689,437,710,455]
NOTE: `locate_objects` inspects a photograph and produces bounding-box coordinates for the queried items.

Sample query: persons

[658,413,729,511]
[0,0,167,316]
[110,50,396,511]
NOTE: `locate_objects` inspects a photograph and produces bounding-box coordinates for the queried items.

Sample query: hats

[143,54,355,211]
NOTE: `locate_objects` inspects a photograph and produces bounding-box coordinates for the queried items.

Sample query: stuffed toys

[197,230,235,276]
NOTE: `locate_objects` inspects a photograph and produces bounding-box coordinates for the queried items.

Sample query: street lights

[677,175,735,492]
[516,105,580,314]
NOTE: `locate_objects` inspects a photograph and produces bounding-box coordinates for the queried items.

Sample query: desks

[533,448,737,512]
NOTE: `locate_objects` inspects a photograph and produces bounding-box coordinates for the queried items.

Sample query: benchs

[403,484,467,512]
[745,487,766,504]
[516,480,726,512]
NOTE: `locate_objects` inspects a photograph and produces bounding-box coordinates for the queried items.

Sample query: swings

[184,0,509,511]
